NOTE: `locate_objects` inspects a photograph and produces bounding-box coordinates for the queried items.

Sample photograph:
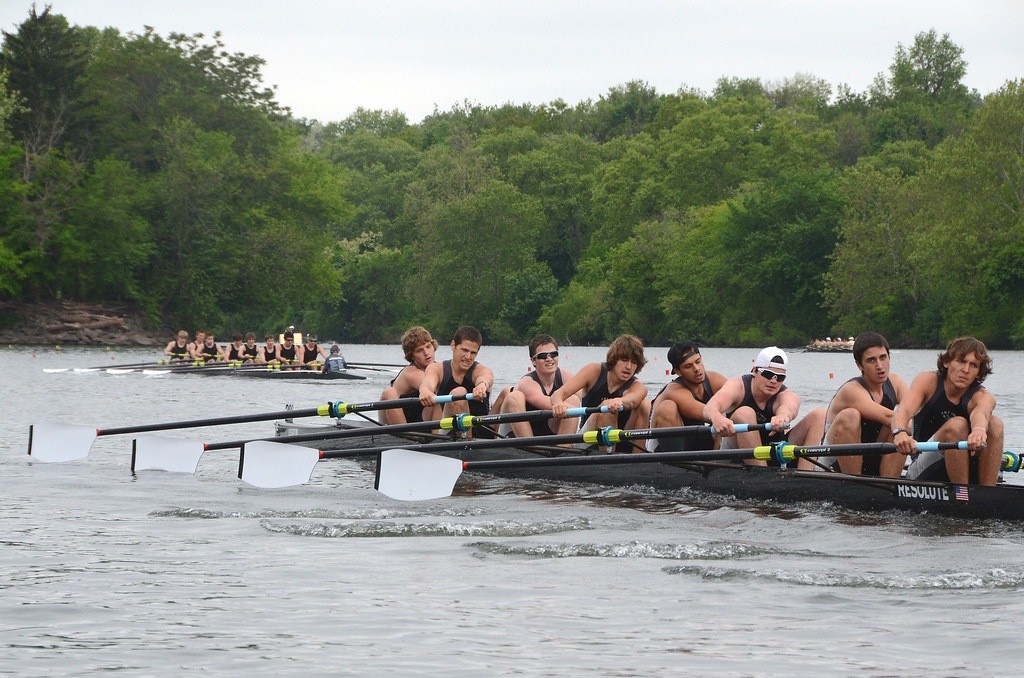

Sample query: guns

[43,353,408,375]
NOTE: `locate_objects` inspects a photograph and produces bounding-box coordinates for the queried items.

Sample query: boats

[164,362,364,380]
[279,423,1021,523]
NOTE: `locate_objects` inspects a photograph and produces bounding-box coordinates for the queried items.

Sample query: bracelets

[476,380,486,385]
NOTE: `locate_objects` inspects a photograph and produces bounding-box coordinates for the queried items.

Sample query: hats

[306,333,318,340]
[837,338,841,340]
[849,337,854,340]
[667,341,699,375]
[826,337,831,341]
[751,347,787,372]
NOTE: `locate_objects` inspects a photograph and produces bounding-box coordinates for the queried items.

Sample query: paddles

[238,423,791,488]
[374,441,987,501]
[27,393,487,463]
[130,405,624,471]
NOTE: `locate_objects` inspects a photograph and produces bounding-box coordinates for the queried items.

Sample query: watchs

[891,427,908,438]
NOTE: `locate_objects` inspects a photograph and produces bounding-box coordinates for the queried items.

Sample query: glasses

[286,339,293,341]
[532,350,559,359]
[758,368,786,382]
[309,340,317,343]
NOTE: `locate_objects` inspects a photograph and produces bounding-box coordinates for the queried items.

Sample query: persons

[501,334,584,448]
[419,327,509,440]
[890,338,1004,486]
[702,345,827,472]
[813,331,913,479]
[648,340,728,450]
[164,328,348,374]
[379,326,441,429]
[811,337,855,350]
[550,335,651,456]
